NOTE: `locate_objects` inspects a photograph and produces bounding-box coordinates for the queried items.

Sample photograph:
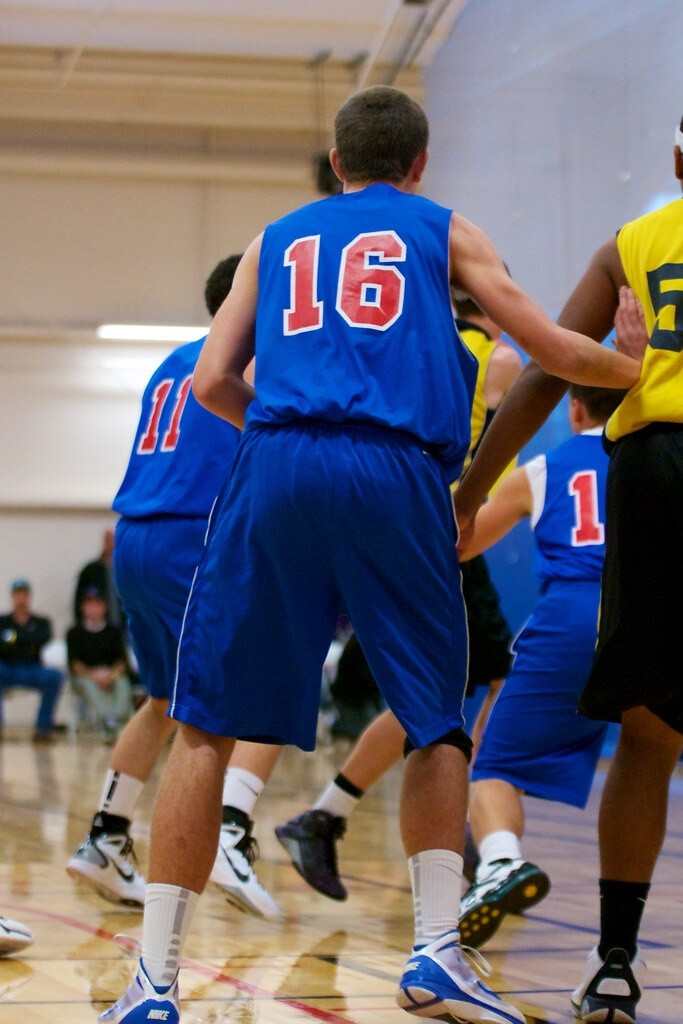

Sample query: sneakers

[207,820,282,923]
[463,823,523,916]
[457,855,551,954]
[97,933,182,1024]
[275,809,347,901]
[66,831,145,909]
[569,942,648,1024]
[395,929,527,1024]
[0,915,33,956]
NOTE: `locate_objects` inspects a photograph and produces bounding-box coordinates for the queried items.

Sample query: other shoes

[33,730,56,744]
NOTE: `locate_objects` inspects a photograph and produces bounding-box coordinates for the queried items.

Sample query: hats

[80,583,104,600]
[11,578,30,591]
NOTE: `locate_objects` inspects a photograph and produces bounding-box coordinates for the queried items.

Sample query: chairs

[67,682,147,744]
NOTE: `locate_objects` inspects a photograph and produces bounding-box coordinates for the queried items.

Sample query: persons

[0,85,683,1024]
